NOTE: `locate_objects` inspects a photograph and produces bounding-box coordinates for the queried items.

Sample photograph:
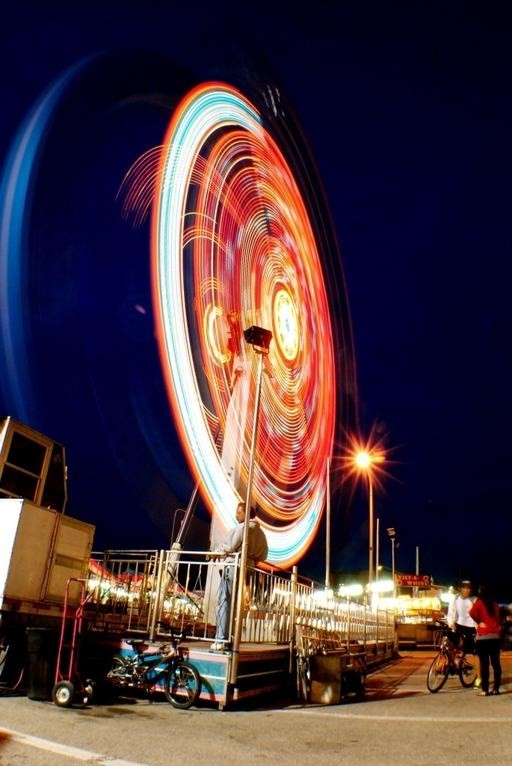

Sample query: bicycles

[426,621,479,692]
[106,620,201,708]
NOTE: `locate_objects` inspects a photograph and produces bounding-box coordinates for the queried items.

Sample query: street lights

[354,446,375,585]
[387,527,396,585]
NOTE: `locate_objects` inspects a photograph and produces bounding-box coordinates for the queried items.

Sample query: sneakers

[211,644,229,651]
[490,689,499,695]
[477,691,488,696]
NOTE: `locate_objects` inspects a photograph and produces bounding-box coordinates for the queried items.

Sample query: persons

[204,501,269,651]
[440,580,483,691]
[469,581,503,697]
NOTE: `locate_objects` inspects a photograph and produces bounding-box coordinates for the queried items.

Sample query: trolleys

[51,578,96,707]
